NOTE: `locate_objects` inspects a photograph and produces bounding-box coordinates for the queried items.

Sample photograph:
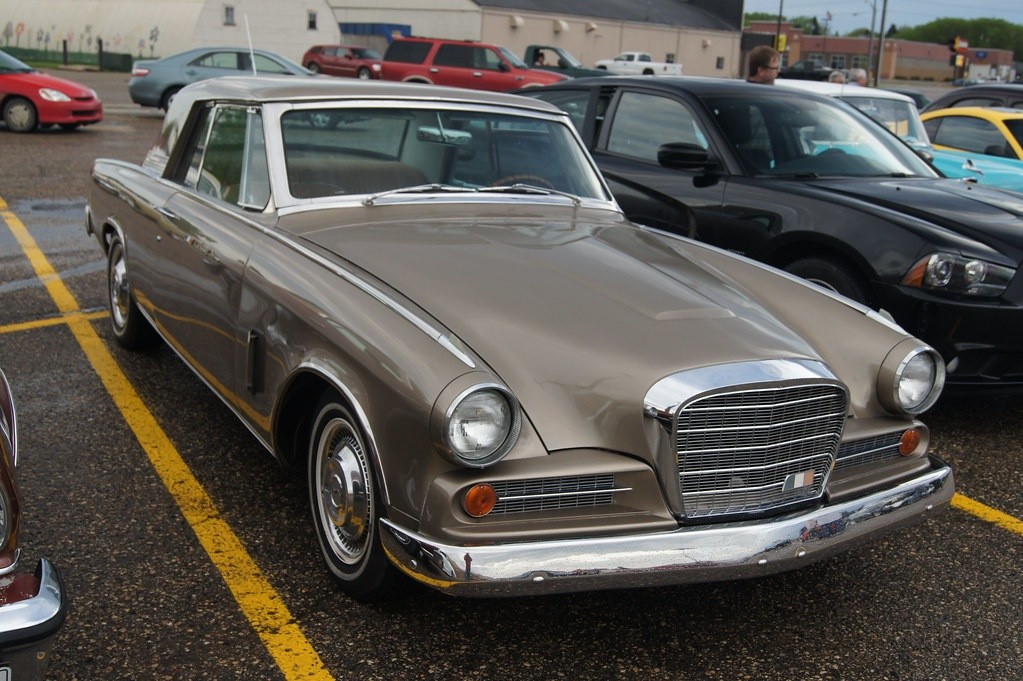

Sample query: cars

[302,43,383,80]
[779,59,833,81]
[441,75,1023,414]
[80,75,956,609]
[687,76,1023,197]
[0,48,103,133]
[128,44,373,131]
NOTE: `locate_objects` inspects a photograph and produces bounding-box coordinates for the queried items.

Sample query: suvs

[378,35,576,108]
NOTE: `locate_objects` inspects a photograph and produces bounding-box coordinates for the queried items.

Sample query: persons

[829,69,846,86]
[846,68,869,87]
[745,44,781,86]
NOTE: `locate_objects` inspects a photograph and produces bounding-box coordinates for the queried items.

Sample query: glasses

[764,65,779,73]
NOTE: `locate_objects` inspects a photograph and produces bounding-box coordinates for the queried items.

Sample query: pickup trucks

[484,43,619,80]
[593,50,683,76]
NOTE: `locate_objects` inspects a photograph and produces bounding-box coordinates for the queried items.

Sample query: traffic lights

[948,36,959,53]
[950,55,964,68]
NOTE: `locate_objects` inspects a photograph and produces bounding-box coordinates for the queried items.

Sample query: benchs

[280,158,429,196]
